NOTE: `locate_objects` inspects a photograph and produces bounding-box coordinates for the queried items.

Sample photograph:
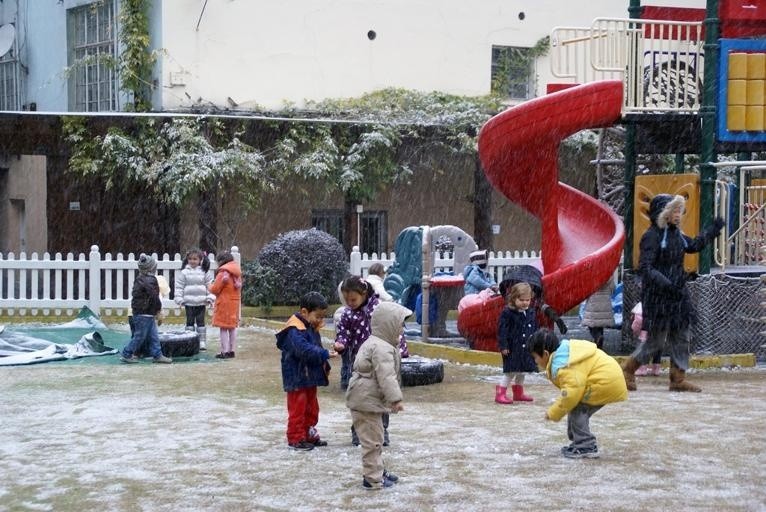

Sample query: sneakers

[153,355,171,362]
[288,440,327,451]
[216,351,235,358]
[120,355,139,362]
[363,469,398,489]
[562,445,598,459]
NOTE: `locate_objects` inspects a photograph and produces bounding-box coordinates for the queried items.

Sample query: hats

[138,253,157,273]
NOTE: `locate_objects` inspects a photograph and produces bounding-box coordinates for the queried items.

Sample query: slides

[457,81,624,353]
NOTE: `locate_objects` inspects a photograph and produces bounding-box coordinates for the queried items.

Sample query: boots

[197,327,207,350]
[541,304,568,334]
[647,364,659,375]
[669,367,700,392]
[635,365,647,376]
[512,385,533,401]
[621,357,637,391]
[495,385,513,404]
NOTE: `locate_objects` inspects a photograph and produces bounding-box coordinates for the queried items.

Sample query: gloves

[669,285,687,301]
[713,218,726,237]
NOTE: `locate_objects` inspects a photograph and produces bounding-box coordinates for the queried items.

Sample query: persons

[207,251,242,359]
[619,192,725,394]
[275,263,415,489]
[581,275,615,349]
[121,252,173,363]
[128,275,171,357]
[630,278,661,377]
[526,326,629,458]
[463,251,567,405]
[175,249,215,350]
